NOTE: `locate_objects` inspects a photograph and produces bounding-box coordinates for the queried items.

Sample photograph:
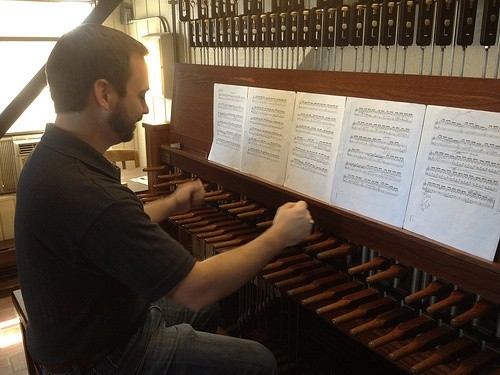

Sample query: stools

[11,288,44,375]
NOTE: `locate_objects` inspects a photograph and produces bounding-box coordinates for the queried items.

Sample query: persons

[14,24,314,375]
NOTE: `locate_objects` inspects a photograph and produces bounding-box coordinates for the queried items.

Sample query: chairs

[105,150,141,169]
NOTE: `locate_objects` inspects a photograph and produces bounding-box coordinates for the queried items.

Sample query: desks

[121,166,149,193]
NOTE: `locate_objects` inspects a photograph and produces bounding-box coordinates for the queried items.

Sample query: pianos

[133,61,500,375]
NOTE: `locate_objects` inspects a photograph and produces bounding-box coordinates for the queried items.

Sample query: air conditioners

[1,135,40,191]
